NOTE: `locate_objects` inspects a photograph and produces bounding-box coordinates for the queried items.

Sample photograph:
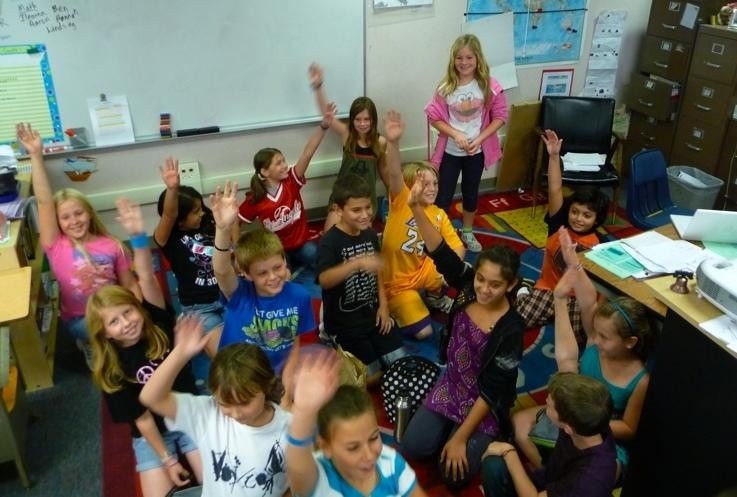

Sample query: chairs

[533,96,621,225]
[627,149,697,228]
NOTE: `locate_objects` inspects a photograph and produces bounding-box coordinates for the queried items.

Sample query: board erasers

[176,128,198,137]
[199,126,219,133]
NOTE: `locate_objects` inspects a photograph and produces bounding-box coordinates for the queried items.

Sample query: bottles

[394,387,412,446]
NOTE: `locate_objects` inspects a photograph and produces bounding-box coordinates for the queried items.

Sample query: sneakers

[461,230,482,253]
[76,339,98,372]
[504,277,535,290]
[424,289,455,315]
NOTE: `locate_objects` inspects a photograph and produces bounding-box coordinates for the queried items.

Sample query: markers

[64,128,87,145]
[42,145,68,154]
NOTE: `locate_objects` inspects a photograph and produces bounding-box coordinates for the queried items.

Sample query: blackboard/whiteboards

[0,0,367,161]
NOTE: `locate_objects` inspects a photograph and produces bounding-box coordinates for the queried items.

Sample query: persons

[306,60,386,233]
[84,101,658,497]
[423,34,508,253]
[14,120,142,373]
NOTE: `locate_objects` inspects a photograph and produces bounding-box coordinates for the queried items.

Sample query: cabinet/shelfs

[671,24,737,174]
[627,0,694,161]
[1,217,59,394]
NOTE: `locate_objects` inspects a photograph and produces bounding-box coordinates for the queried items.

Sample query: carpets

[103,347,485,496]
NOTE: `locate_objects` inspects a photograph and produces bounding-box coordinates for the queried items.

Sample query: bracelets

[311,80,323,89]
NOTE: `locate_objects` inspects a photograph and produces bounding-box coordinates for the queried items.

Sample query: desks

[580,209,737,479]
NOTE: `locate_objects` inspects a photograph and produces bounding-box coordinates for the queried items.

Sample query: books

[0,144,25,219]
[16,201,52,333]
